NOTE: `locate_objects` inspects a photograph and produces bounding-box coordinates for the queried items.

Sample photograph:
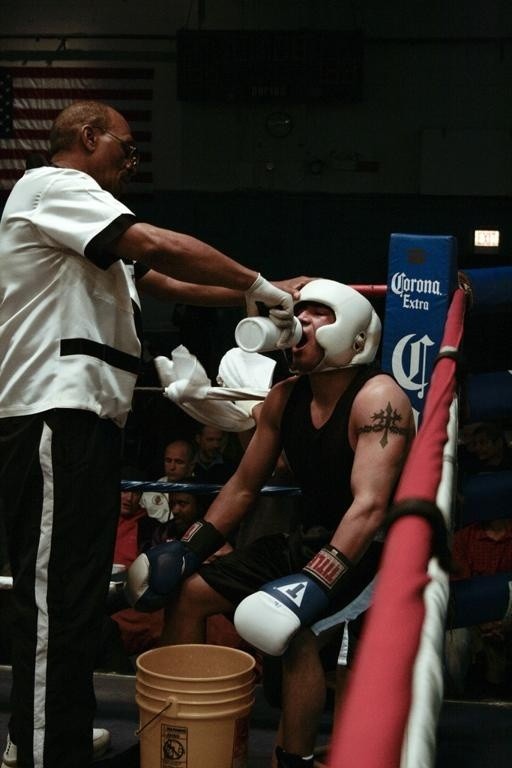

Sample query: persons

[101,276,511,768]
[0,99,317,767]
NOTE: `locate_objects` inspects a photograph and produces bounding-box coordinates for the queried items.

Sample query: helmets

[283,279,383,367]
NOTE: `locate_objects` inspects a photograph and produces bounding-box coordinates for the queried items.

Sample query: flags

[0,49,158,210]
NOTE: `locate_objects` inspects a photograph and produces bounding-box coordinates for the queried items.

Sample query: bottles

[235,313,306,354]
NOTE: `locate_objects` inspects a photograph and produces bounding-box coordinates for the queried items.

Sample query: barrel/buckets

[135,644,256,768]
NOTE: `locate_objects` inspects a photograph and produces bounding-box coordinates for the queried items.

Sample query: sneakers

[0,728,111,768]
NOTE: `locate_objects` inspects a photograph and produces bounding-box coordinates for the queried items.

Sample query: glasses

[93,124,138,168]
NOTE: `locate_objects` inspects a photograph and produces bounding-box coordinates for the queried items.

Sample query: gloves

[243,273,297,350]
[123,520,226,612]
[234,545,352,657]
